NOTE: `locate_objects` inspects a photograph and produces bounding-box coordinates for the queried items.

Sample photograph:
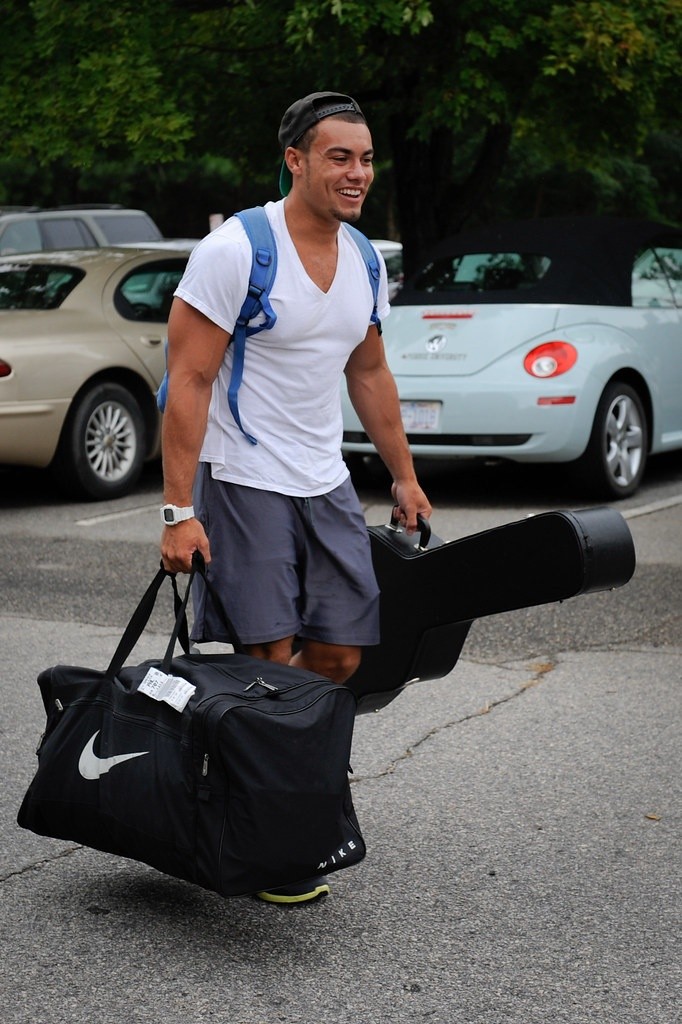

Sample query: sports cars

[317,224,680,500]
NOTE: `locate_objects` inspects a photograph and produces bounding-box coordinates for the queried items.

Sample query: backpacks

[157,208,381,416]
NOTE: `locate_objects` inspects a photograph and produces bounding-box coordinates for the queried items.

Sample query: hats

[278,92,363,199]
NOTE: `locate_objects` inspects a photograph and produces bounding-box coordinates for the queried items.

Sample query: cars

[0,198,162,263]
[0,242,199,496]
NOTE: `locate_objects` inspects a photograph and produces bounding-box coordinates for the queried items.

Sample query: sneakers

[258,877,329,907]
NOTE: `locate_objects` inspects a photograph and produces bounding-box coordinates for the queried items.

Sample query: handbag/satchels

[18,546,367,898]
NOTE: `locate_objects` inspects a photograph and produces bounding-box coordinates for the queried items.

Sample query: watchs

[161,503,195,525]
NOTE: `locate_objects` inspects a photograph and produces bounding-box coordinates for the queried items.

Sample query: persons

[160,90,432,906]
